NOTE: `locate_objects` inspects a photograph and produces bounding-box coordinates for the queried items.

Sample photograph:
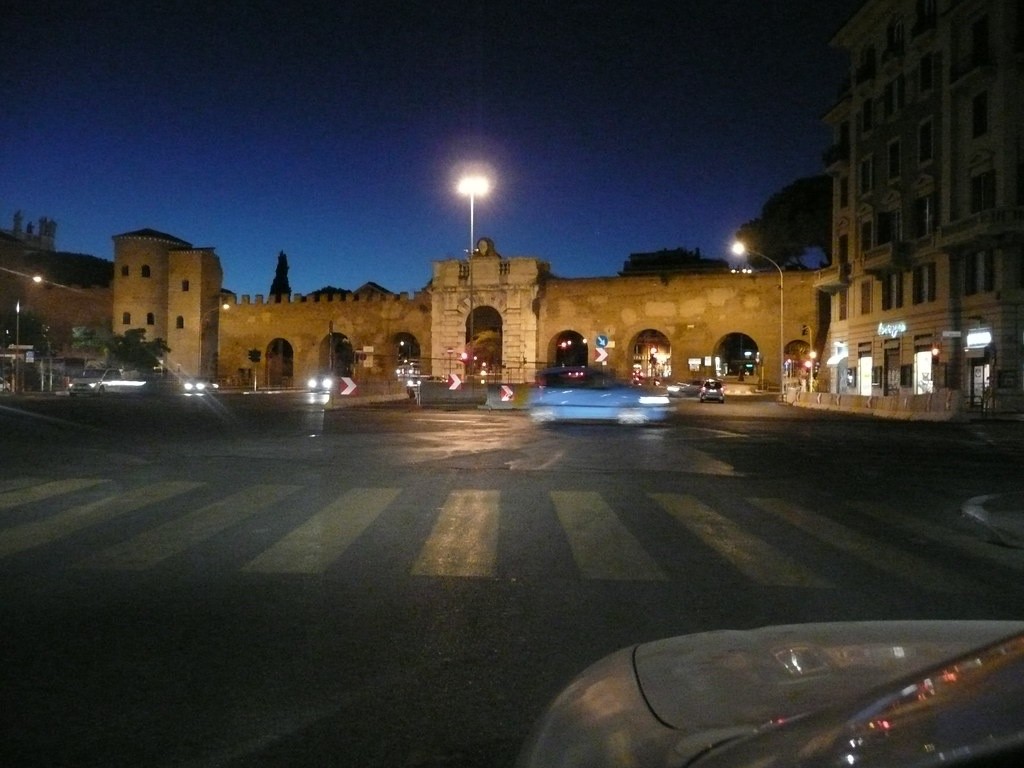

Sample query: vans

[69,368,122,396]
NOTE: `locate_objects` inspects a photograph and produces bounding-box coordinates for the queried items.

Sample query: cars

[527,366,676,425]
[308,370,333,392]
[0,378,11,392]
[186,376,218,394]
[523,619,1023,768]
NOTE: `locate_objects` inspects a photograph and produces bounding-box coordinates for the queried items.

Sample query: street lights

[14,272,43,393]
[451,167,496,355]
[198,304,230,376]
[730,241,785,394]
[803,326,818,390]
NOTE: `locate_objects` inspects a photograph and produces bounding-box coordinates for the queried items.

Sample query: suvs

[700,380,725,403]
